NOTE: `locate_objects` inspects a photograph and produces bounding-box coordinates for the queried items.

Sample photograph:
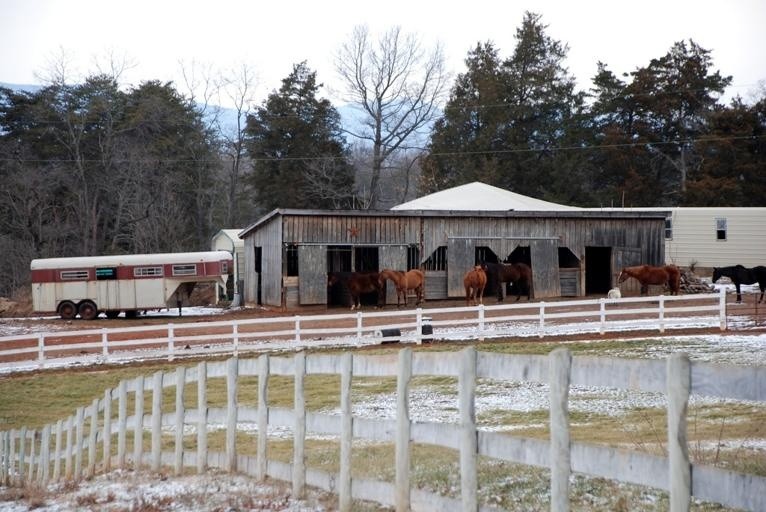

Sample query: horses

[328,271,385,311]
[480,258,533,304]
[378,268,424,310]
[712,265,766,304]
[464,264,489,306]
[618,264,680,296]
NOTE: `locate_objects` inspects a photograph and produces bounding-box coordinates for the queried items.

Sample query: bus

[29,250,234,320]
